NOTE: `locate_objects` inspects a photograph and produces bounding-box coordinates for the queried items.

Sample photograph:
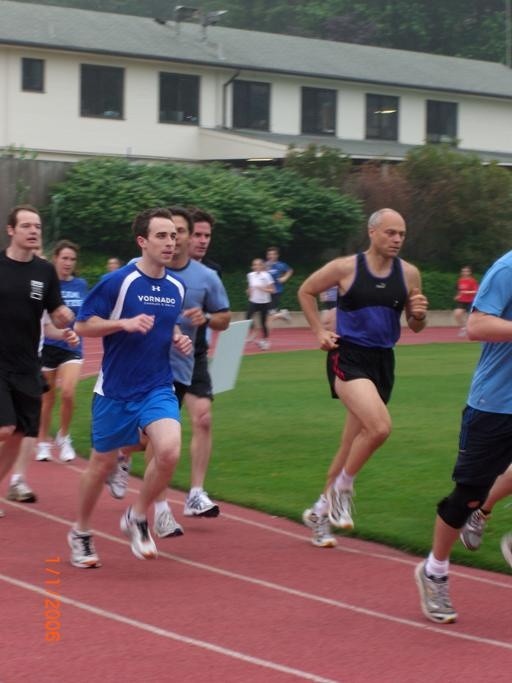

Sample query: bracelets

[412,314,425,321]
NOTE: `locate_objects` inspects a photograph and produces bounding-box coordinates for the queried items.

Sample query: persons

[454,266,479,337]
[319,248,338,333]
[116,206,232,538]
[102,210,220,518]
[0,206,75,519]
[5,248,79,502]
[414,248,512,623]
[66,209,192,568]
[262,247,293,350]
[245,259,276,347]
[32,241,87,462]
[296,208,428,547]
[108,257,121,272]
[460,464,512,551]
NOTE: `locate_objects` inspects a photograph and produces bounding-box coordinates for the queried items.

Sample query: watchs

[205,312,212,325]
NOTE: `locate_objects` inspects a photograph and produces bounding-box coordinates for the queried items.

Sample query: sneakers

[500,532,511,567]
[460,508,491,551]
[414,560,457,622]
[56,430,76,462]
[183,491,219,518]
[119,504,158,559]
[327,484,355,529]
[66,528,102,568]
[153,512,185,538]
[35,442,51,461]
[280,309,289,323]
[303,504,338,548]
[108,459,129,500]
[7,479,37,502]
